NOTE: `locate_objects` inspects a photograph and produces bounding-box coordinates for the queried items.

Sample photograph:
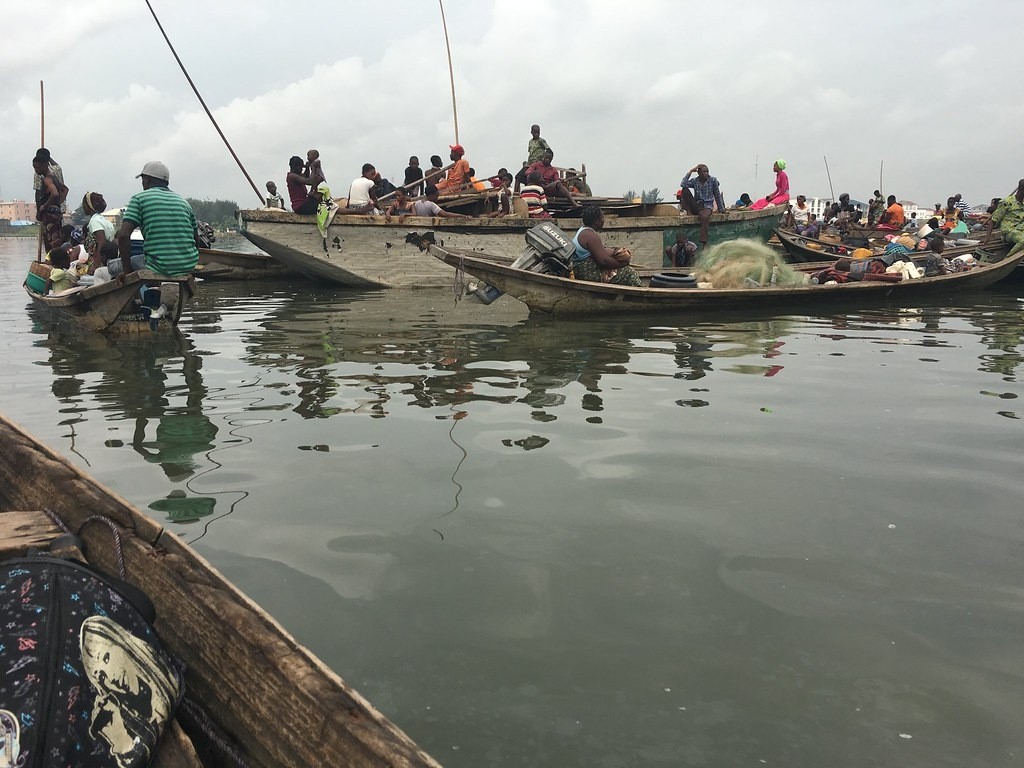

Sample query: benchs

[0,510,67,560]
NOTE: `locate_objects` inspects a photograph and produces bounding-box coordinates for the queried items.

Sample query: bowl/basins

[26,271,50,294]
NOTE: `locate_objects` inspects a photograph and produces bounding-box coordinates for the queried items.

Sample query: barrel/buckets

[973,246,997,264]
[917,224,933,239]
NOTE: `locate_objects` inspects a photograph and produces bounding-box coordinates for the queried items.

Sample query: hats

[934,203,941,207]
[839,193,849,200]
[134,161,170,182]
[776,159,786,170]
[674,190,683,195]
[71,229,82,241]
[448,144,465,157]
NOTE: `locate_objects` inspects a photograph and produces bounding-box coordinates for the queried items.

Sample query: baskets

[30,260,53,290]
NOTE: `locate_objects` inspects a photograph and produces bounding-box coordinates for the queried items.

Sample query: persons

[738,159,790,211]
[263,181,286,210]
[740,194,753,211]
[528,125,550,165]
[783,190,1002,235]
[32,148,118,297]
[287,145,592,223]
[666,232,697,267]
[924,238,952,277]
[713,181,725,212]
[980,179,1024,258]
[674,190,682,210]
[572,205,641,287]
[107,160,200,285]
[680,164,731,250]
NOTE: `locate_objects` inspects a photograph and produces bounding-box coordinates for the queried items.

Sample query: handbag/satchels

[1,549,189,768]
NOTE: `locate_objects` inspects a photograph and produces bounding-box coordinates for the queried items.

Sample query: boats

[405,228,1024,314]
[769,221,1005,262]
[116,237,291,282]
[24,252,197,333]
[233,204,791,287]
